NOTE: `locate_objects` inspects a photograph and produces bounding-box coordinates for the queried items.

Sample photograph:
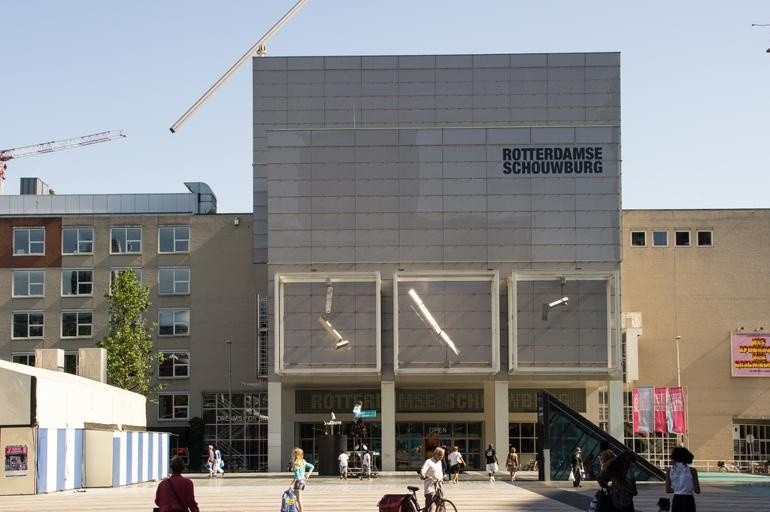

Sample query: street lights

[227,336,237,470]
[674,334,682,445]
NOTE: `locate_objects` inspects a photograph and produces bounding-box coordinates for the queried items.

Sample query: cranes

[0,127,131,195]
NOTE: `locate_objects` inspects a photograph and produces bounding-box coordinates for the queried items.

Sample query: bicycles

[377,473,457,512]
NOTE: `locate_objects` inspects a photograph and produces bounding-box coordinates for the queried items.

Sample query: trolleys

[281,487,299,512]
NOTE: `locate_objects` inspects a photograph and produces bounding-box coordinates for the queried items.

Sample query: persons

[420,446,445,512]
[338,450,350,480]
[353,400,363,424]
[506,447,518,482]
[360,450,372,480]
[441,445,451,480]
[207,445,214,477]
[485,443,498,482]
[449,446,466,484]
[213,446,224,477]
[570,441,638,512]
[290,448,314,512]
[155,455,200,512]
[665,448,700,512]
